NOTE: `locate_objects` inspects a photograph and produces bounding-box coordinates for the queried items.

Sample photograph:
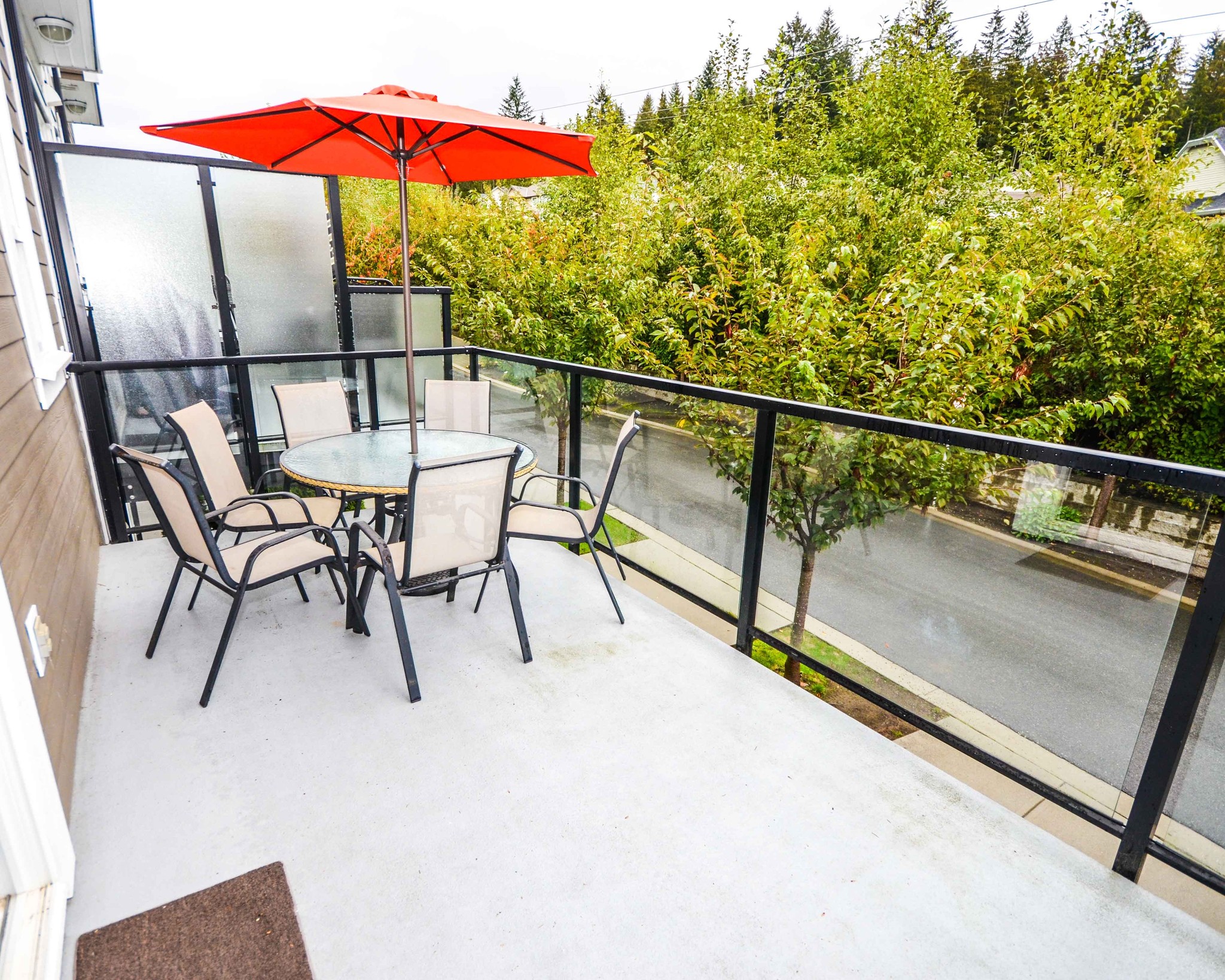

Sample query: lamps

[44,82,64,107]
[64,100,87,116]
[33,16,74,45]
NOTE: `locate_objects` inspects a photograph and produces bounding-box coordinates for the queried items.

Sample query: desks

[280,428,539,631]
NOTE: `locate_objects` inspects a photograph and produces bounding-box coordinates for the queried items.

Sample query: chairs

[346,443,534,704]
[421,379,493,434]
[161,399,357,605]
[472,408,645,625]
[270,379,392,570]
[109,442,370,709]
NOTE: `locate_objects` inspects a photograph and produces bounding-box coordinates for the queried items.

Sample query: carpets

[72,861,315,980]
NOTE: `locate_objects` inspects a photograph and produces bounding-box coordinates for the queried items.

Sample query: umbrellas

[140,87,599,454]
